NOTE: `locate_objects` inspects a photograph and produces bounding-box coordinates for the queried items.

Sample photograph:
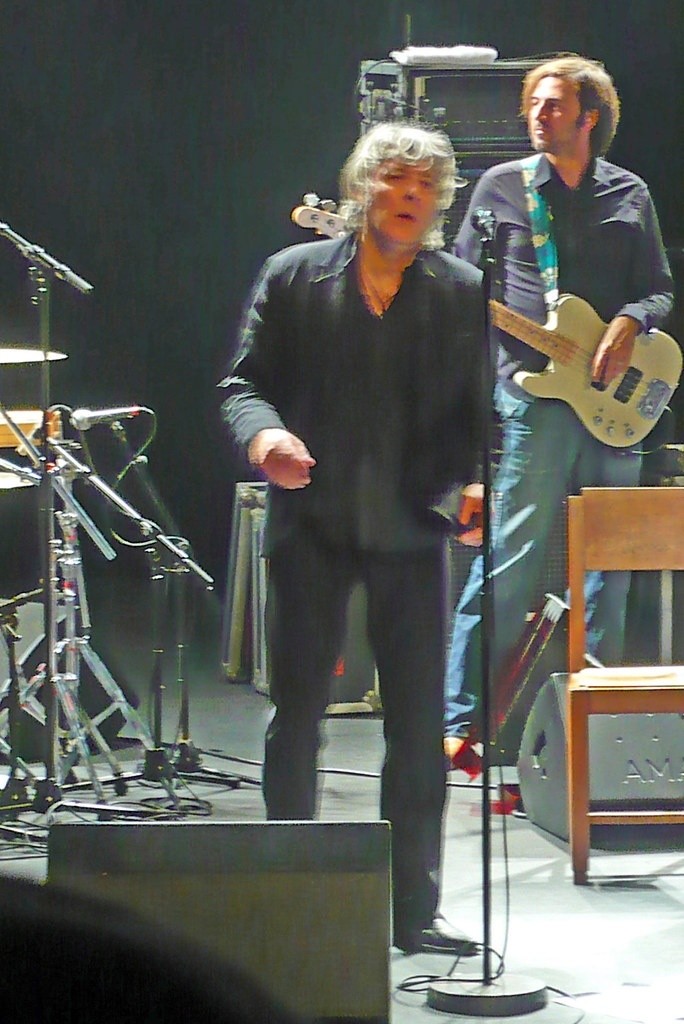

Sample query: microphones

[70,406,146,431]
[470,206,496,240]
[17,421,44,456]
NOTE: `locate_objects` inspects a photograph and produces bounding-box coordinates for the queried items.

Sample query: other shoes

[439,738,469,770]
[395,925,478,958]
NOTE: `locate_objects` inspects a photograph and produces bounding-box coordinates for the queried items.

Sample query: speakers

[516,672,684,841]
[43,822,393,1024]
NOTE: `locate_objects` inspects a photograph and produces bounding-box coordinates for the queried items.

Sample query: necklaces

[363,268,399,314]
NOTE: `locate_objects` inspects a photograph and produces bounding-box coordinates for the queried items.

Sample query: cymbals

[2,347,69,368]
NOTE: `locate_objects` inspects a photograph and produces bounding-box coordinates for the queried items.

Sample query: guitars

[290,190,683,450]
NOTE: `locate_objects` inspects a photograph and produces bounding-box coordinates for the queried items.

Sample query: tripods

[0,221,262,822]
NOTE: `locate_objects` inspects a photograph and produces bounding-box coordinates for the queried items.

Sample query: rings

[616,362,624,365]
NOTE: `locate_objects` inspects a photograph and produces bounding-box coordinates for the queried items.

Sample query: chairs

[566,487,684,886]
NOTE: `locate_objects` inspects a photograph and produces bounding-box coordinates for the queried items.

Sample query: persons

[211,121,496,954]
[444,55,677,771]
